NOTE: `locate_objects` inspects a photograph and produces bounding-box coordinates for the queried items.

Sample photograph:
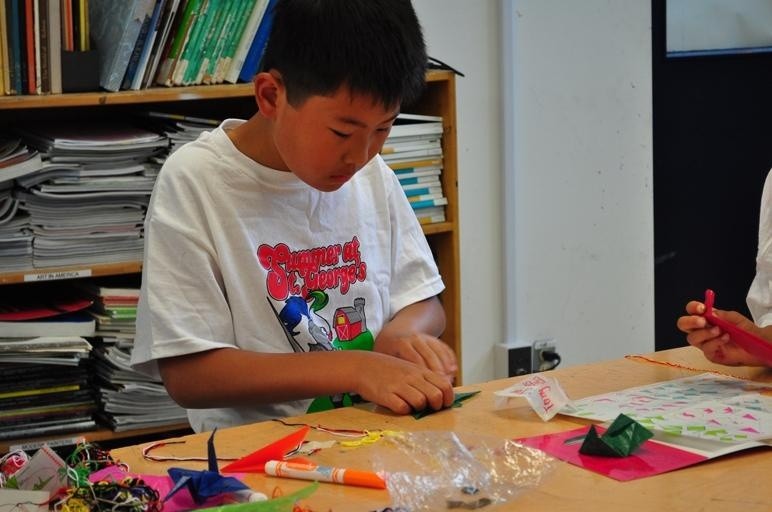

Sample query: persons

[675,164,771,369]
[126,2,458,437]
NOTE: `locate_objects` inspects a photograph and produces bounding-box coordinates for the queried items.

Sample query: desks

[106,320,772,512]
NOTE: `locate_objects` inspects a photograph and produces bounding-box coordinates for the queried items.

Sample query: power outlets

[531,339,557,374]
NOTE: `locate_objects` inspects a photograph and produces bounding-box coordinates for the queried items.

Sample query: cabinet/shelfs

[0,51,462,467]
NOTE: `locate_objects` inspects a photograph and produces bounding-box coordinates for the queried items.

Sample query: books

[381,114,447,225]
[0,1,276,440]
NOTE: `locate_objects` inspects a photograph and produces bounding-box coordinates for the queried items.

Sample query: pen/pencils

[265,460,387,488]
